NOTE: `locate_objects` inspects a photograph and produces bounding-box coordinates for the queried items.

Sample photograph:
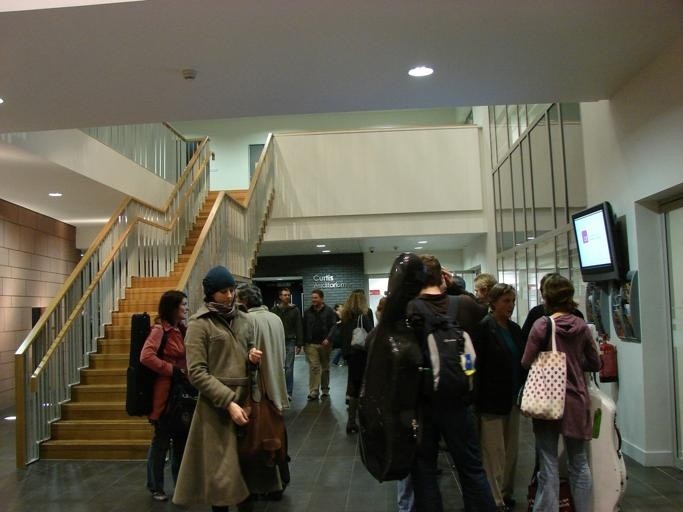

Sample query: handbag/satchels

[238,351,288,465]
[519,315,567,420]
[351,313,368,349]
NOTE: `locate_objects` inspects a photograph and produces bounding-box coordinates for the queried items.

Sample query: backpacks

[404,295,478,407]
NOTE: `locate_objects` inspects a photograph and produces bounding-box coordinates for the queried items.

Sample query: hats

[202,265,235,296]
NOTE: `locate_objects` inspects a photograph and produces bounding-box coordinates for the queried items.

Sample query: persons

[233,283,291,501]
[302,290,336,400]
[451,275,466,291]
[521,275,602,512]
[478,283,521,511]
[375,297,386,323]
[172,266,263,511]
[269,288,302,401]
[338,289,374,436]
[521,273,584,353]
[331,303,344,365]
[396,256,496,512]
[139,290,190,502]
[474,271,498,312]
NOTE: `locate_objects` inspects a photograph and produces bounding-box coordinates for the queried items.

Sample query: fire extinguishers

[596,332,617,384]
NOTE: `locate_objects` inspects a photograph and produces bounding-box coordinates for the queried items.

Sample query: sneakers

[151,491,168,501]
[307,390,319,400]
[321,387,330,400]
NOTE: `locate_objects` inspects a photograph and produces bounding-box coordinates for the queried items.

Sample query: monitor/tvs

[570,200,621,282]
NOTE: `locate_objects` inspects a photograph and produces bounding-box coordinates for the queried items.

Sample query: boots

[346,397,359,434]
